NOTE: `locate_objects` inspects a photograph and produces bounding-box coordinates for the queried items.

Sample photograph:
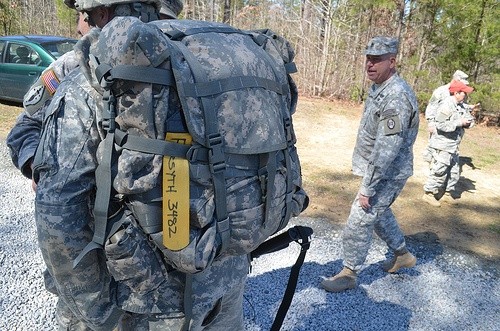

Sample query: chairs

[16,47,32,64]
[11,46,20,62]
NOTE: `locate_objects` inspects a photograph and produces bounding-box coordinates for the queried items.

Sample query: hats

[452,70,470,85]
[157,0,183,20]
[65,1,160,12]
[448,80,474,94]
[364,36,401,58]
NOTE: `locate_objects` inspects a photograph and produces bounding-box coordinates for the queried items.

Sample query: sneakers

[380,248,416,273]
[423,191,442,209]
[321,264,359,294]
[438,188,458,206]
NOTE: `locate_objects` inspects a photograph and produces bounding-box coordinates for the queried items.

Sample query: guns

[249,225,314,260]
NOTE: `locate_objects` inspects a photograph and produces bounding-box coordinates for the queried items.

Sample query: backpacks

[86,16,309,268]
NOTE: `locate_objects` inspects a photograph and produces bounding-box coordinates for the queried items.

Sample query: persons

[29,0,247,330]
[425,69,482,186]
[316,38,418,292]
[423,80,473,208]
[5,0,186,328]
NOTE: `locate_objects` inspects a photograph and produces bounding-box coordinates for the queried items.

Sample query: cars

[0,35,82,105]
